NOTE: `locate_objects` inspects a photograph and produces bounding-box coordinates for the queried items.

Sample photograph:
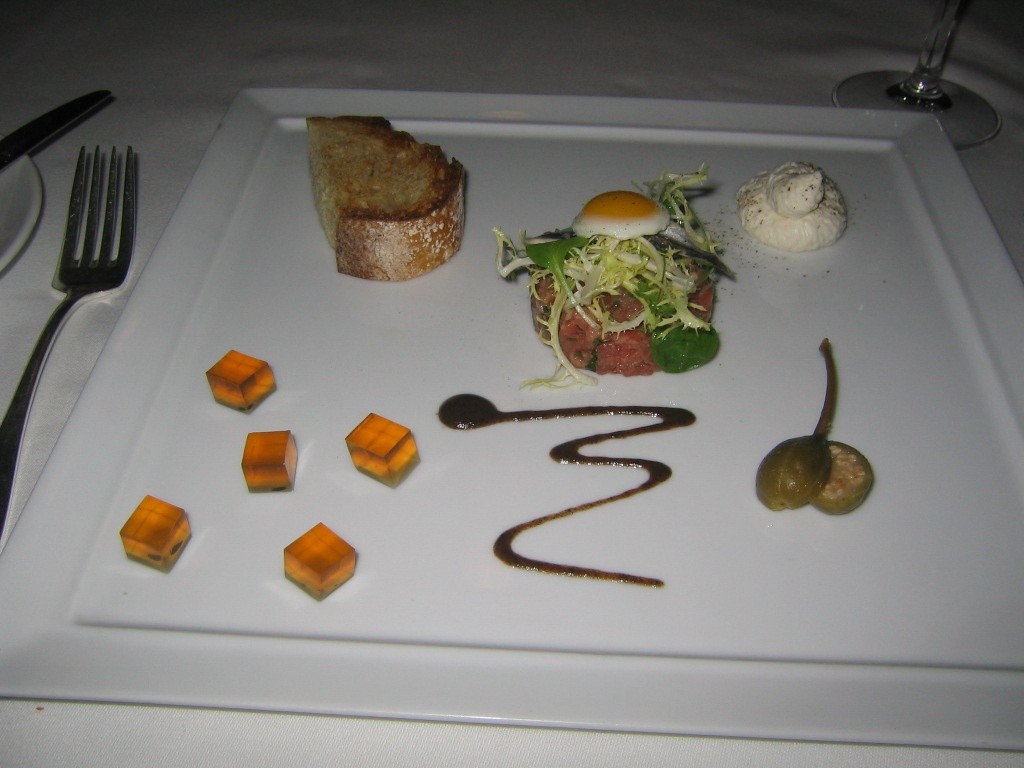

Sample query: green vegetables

[495,161,738,386]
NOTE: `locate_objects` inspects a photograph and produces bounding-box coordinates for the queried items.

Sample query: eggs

[573,190,673,238]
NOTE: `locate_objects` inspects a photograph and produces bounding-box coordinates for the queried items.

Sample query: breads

[304,114,464,281]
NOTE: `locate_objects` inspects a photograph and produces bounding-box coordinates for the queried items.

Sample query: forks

[0,146,135,540]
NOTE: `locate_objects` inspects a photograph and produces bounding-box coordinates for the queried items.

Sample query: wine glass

[832,0,1002,149]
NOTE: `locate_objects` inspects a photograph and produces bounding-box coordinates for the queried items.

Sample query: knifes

[0,89,112,174]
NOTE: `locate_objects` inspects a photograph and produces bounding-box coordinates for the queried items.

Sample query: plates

[1,132,43,275]
[0,86,1023,752]
